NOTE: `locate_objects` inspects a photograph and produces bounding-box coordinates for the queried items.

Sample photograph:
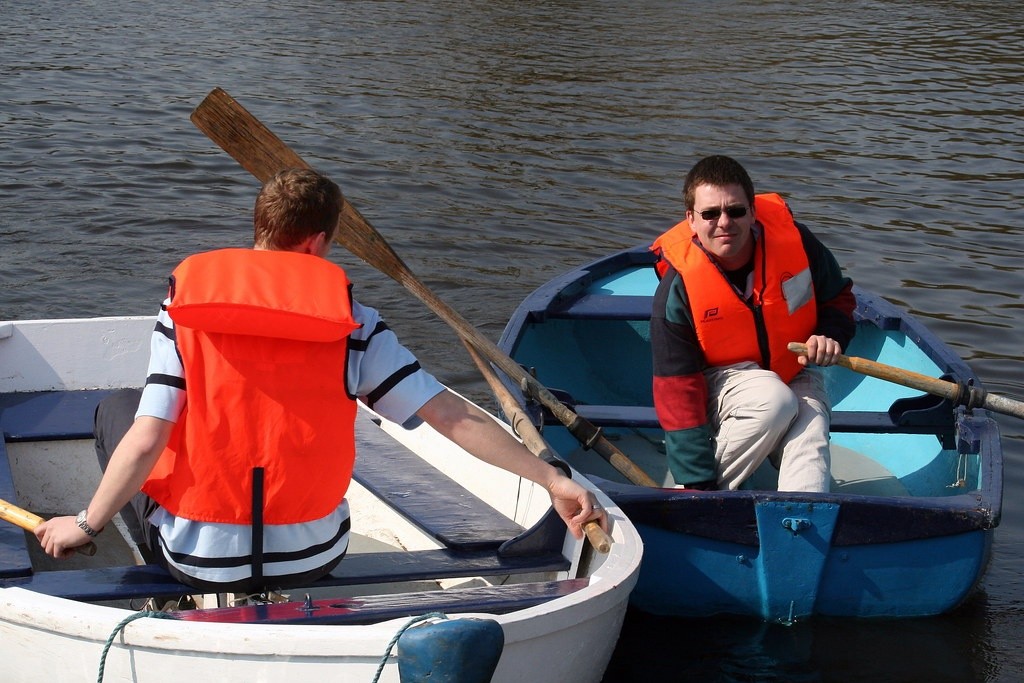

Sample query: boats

[0,315,644,682]
[490,242,1005,628]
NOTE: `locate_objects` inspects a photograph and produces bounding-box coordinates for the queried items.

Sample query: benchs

[550,293,655,322]
[0,389,118,443]
[0,547,573,602]
[0,430,34,576]
[516,364,962,450]
[353,411,528,549]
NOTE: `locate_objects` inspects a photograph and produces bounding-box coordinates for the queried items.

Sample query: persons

[34,170,609,595]
[650,155,856,494]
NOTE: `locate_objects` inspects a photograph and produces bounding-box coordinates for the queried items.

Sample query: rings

[592,504,601,509]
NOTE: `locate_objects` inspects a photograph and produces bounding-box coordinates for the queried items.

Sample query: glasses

[694,206,752,221]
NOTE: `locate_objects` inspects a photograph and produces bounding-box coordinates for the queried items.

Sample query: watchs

[75,508,105,537]
